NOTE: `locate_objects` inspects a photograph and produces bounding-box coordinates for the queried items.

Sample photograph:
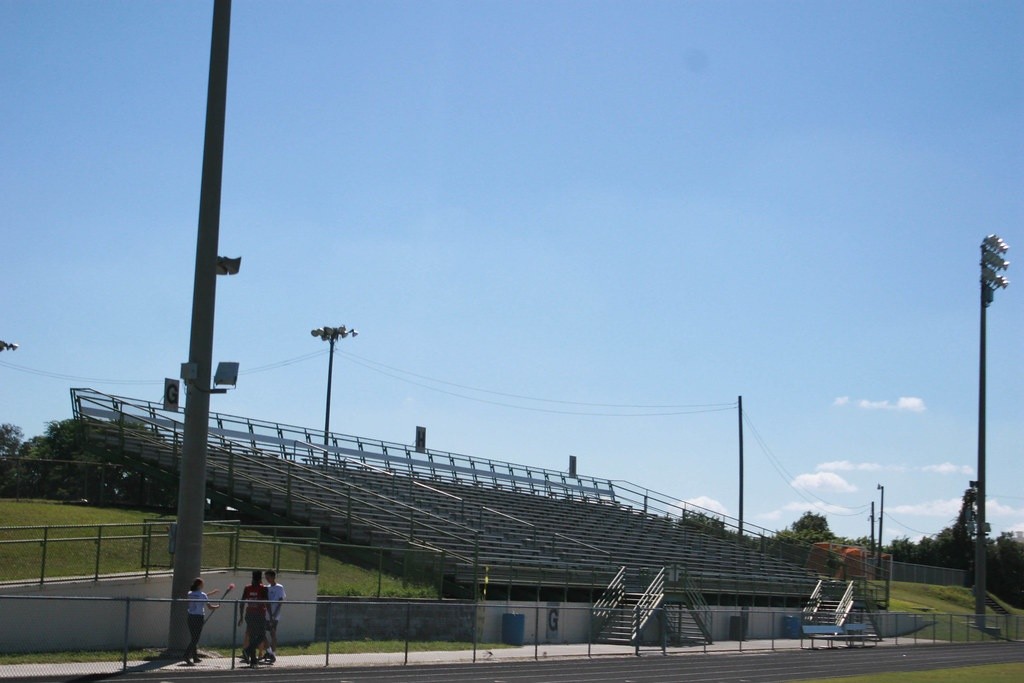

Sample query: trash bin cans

[730,616,746,641]
[784,616,801,639]
[502,613,525,645]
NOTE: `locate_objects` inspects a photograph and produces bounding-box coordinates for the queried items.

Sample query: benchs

[85,421,860,593]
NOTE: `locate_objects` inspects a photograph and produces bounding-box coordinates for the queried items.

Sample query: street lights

[310,324,359,466]
[974,233,1010,627]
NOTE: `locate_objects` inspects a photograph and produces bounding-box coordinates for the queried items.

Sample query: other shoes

[183,656,194,666]
[192,657,202,663]
[256,655,262,660]
[270,653,276,663]
[251,656,258,666]
[262,652,272,659]
[243,648,250,664]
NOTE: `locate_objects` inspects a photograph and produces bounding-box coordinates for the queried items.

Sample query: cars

[63,494,87,505]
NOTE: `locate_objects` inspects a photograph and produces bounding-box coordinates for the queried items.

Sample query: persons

[183,578,220,665]
[237,570,287,664]
